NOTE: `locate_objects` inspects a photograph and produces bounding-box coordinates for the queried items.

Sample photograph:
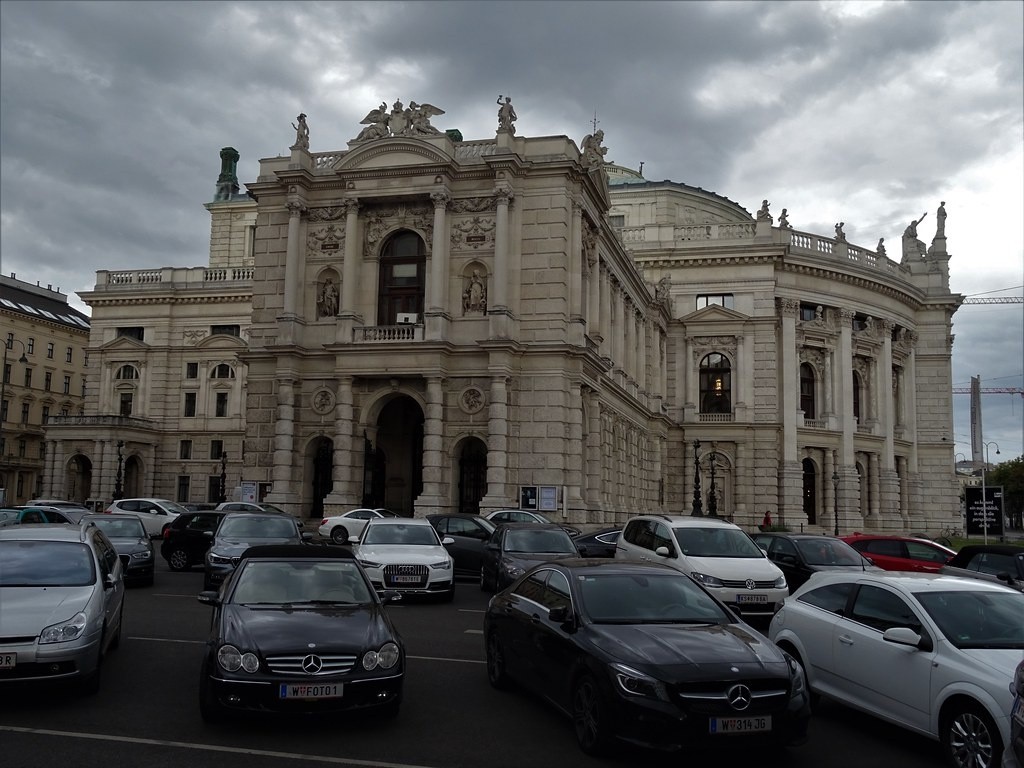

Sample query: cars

[198,512,314,597]
[821,531,976,575]
[194,541,409,730]
[576,523,733,559]
[103,497,200,538]
[1,496,112,531]
[318,508,408,546]
[481,559,815,756]
[426,513,521,583]
[768,569,1024,768]
[354,515,456,606]
[77,513,155,589]
[0,520,127,697]
[937,544,1024,592]
[737,530,888,596]
[216,501,293,517]
[481,519,587,599]
[487,511,583,538]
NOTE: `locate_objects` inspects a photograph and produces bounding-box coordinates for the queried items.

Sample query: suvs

[612,509,792,635]
[160,509,251,574]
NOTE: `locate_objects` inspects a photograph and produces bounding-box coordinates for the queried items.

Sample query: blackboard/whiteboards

[963,485,1006,538]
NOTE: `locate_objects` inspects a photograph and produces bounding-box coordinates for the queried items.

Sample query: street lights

[692,438,704,518]
[705,452,720,517]
[111,440,125,502]
[831,471,840,536]
[0,337,29,432]
[214,451,228,506]
[954,453,966,474]
[982,441,1001,472]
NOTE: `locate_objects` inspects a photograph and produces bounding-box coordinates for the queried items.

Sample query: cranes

[963,285,1024,304]
[951,372,1024,400]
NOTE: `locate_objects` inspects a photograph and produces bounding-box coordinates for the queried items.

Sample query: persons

[835,222,847,242]
[462,269,486,311]
[288,113,309,151]
[317,277,338,316]
[761,199,773,218]
[876,237,887,255]
[580,129,614,187]
[495,94,518,134]
[901,212,927,257]
[935,201,947,237]
[778,208,789,227]
[763,508,772,531]
[349,97,445,142]
